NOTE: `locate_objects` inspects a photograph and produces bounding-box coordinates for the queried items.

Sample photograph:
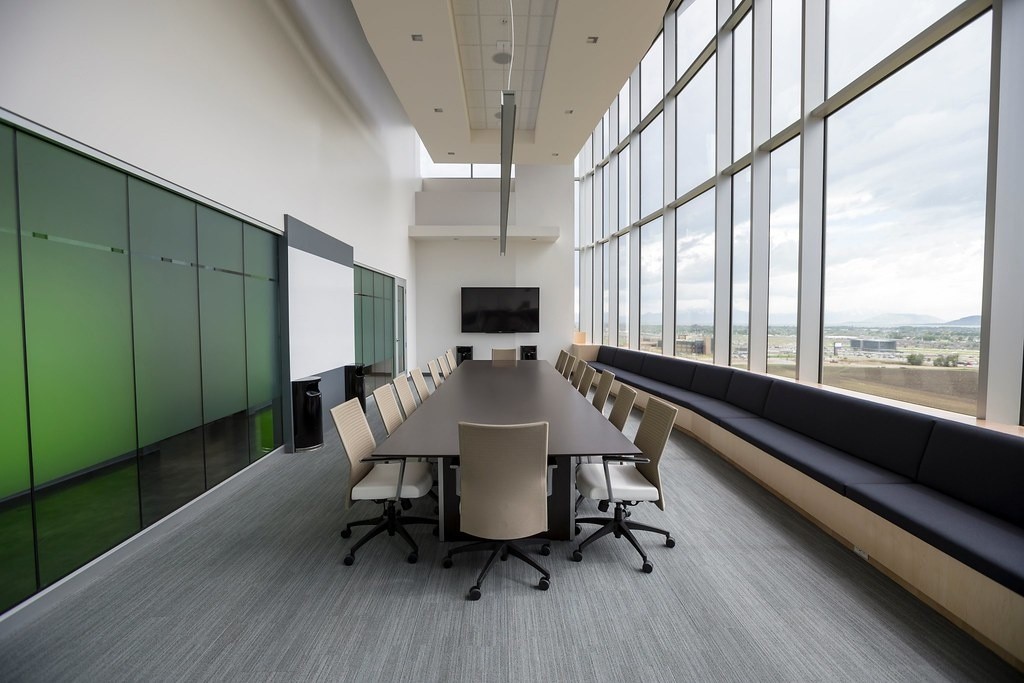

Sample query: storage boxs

[292,376,325,454]
[345,363,366,416]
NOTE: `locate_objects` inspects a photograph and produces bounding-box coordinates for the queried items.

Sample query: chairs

[558,352,568,375]
[491,348,516,360]
[373,384,440,512]
[575,383,652,517]
[436,356,448,381]
[446,352,455,373]
[330,397,433,566]
[575,369,624,467]
[570,359,587,390]
[409,367,430,403]
[427,360,442,390]
[554,350,565,370]
[392,374,428,462]
[576,396,678,574]
[441,422,553,601]
[577,364,596,397]
[563,355,576,381]
[445,348,457,370]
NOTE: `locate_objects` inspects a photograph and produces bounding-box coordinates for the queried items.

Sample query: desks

[372,361,642,543]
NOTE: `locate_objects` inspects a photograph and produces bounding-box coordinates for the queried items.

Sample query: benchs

[583,346,1024,674]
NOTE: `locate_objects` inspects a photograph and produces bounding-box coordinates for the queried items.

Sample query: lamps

[500,2,517,257]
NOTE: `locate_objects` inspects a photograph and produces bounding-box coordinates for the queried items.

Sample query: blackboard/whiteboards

[287,246,356,381]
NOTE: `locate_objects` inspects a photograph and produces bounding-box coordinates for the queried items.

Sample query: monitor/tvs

[460,287,541,333]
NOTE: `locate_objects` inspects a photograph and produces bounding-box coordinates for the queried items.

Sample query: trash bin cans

[345,363,367,414]
[292,375,325,453]
[520,344,537,361]
[456,345,474,367]
